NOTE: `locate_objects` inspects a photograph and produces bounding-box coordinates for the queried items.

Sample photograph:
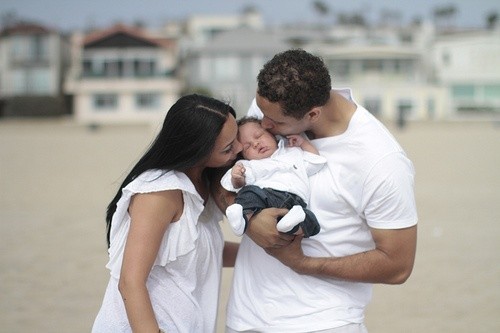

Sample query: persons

[219,116,322,241]
[210,47,419,333]
[88,93,242,333]
